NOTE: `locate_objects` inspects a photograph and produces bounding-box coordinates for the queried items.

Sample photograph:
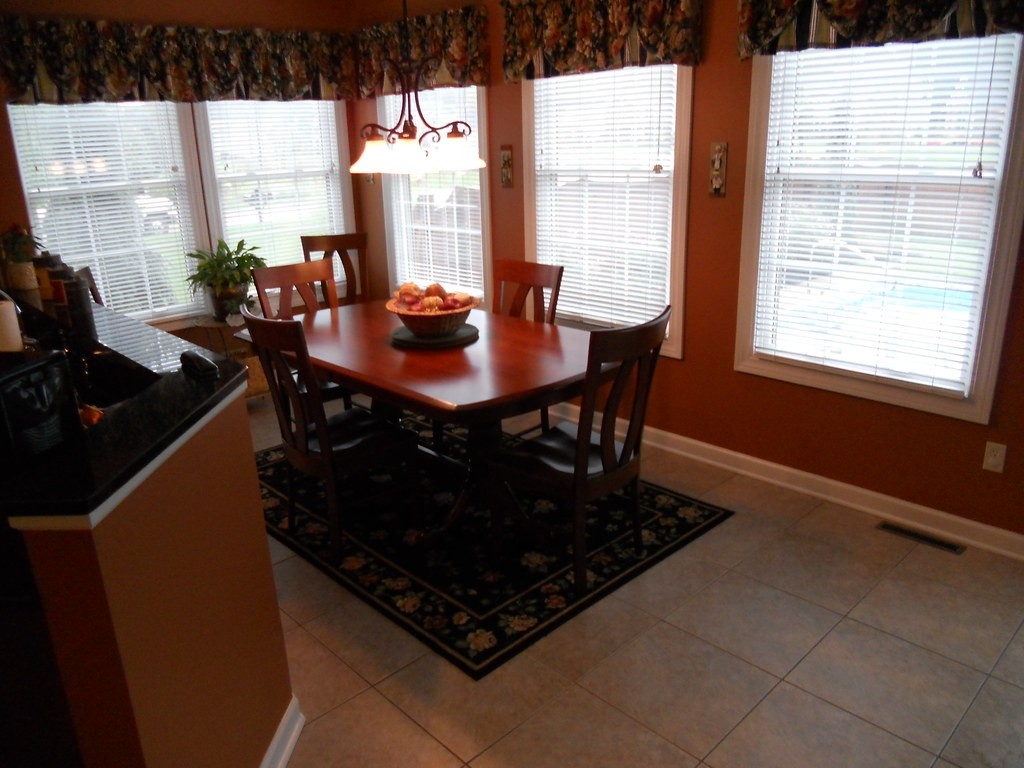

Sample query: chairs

[300,232,368,306]
[239,303,393,553]
[457,258,564,442]
[250,257,360,423]
[479,302,674,584]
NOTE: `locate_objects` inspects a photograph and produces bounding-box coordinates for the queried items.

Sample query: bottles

[32,250,96,335]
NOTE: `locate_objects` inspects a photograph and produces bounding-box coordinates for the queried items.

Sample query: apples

[395,282,470,313]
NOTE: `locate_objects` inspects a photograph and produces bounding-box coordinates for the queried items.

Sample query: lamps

[349,0,486,174]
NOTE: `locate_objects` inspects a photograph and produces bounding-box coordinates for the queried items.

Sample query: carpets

[252,414,737,681]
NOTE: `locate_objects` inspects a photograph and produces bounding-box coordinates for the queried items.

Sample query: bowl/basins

[386,293,482,338]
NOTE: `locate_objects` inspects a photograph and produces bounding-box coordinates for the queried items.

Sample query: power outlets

[982,442,1007,475]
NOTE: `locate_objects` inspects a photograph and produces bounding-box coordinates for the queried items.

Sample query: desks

[193,314,246,357]
[233,301,622,533]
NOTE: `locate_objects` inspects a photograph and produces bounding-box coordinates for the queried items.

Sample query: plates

[390,322,479,347]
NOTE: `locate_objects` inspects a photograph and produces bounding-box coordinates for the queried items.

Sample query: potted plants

[191,241,264,319]
[0,224,45,291]
